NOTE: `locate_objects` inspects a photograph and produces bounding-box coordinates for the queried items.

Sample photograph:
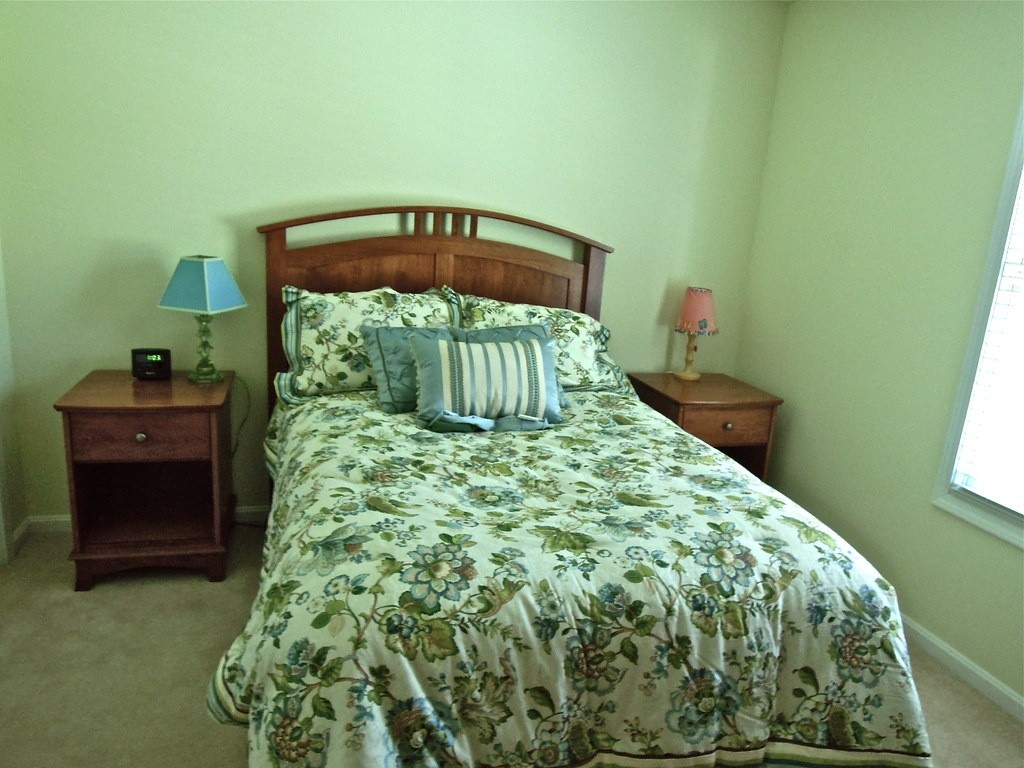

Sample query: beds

[200,204,933,768]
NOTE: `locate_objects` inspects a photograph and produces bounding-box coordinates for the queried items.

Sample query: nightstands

[54,369,238,591]
[628,371,784,482]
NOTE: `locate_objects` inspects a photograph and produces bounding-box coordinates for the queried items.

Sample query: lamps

[157,254,250,384]
[674,287,719,380]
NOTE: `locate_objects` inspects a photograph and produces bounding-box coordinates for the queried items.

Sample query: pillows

[442,286,624,393]
[359,325,456,413]
[409,338,566,433]
[273,284,460,407]
[450,325,569,408]
